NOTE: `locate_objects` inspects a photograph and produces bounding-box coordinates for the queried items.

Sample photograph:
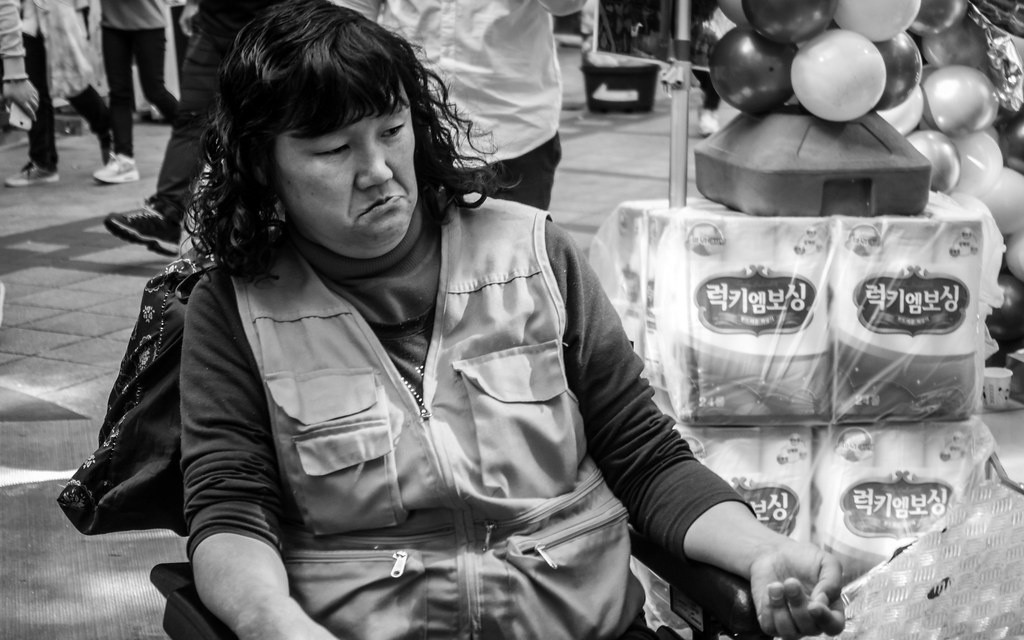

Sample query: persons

[180,0,848,640]
[0,1,588,334]
[672,1,720,134]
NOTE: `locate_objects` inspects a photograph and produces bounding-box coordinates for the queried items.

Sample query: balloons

[707,0,1024,367]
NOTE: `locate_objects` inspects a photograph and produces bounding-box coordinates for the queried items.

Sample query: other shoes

[698,109,719,137]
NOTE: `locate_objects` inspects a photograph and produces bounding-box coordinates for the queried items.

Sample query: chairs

[59,241,773,640]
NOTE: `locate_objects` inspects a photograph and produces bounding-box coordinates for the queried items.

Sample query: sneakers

[92,152,139,182]
[105,207,181,256]
[5,162,59,187]
